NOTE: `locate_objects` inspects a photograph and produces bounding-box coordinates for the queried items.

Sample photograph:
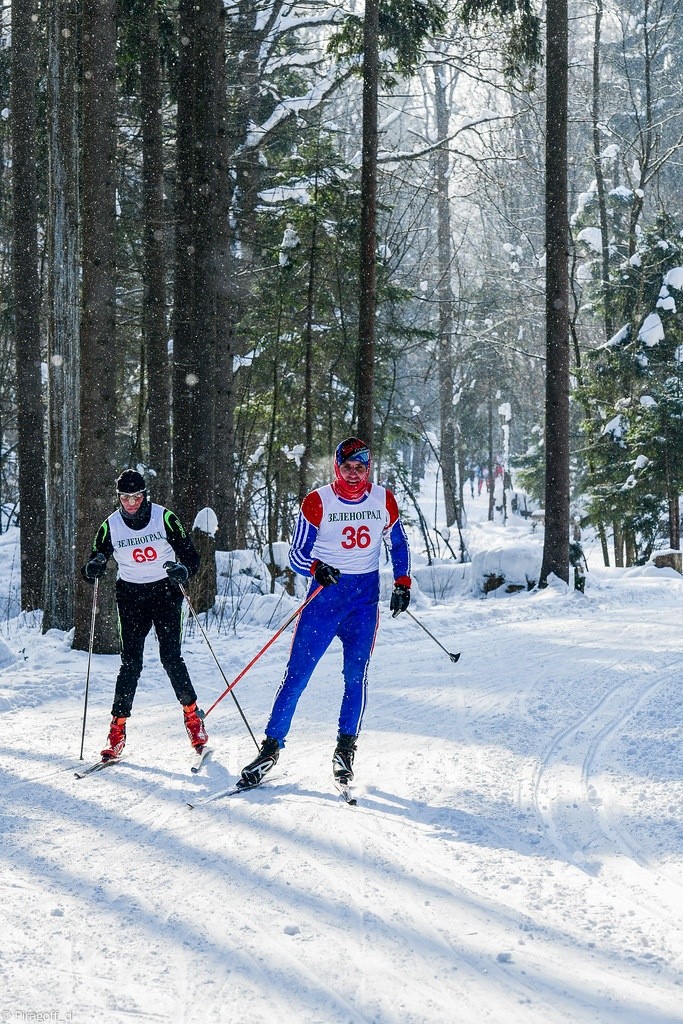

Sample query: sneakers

[183,704,208,747]
[100,717,126,757]
[241,743,279,784]
[333,734,357,781]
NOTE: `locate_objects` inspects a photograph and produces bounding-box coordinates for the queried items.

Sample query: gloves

[85,558,103,578]
[166,561,188,586]
[315,561,340,587]
[390,585,410,618]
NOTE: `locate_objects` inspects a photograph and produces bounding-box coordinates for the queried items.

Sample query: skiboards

[187,769,358,809]
[74,747,213,780]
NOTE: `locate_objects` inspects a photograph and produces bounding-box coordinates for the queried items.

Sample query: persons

[242,436,411,781]
[85,469,210,757]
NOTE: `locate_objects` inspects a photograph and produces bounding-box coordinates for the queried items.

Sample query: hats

[337,438,369,471]
[117,469,146,495]
